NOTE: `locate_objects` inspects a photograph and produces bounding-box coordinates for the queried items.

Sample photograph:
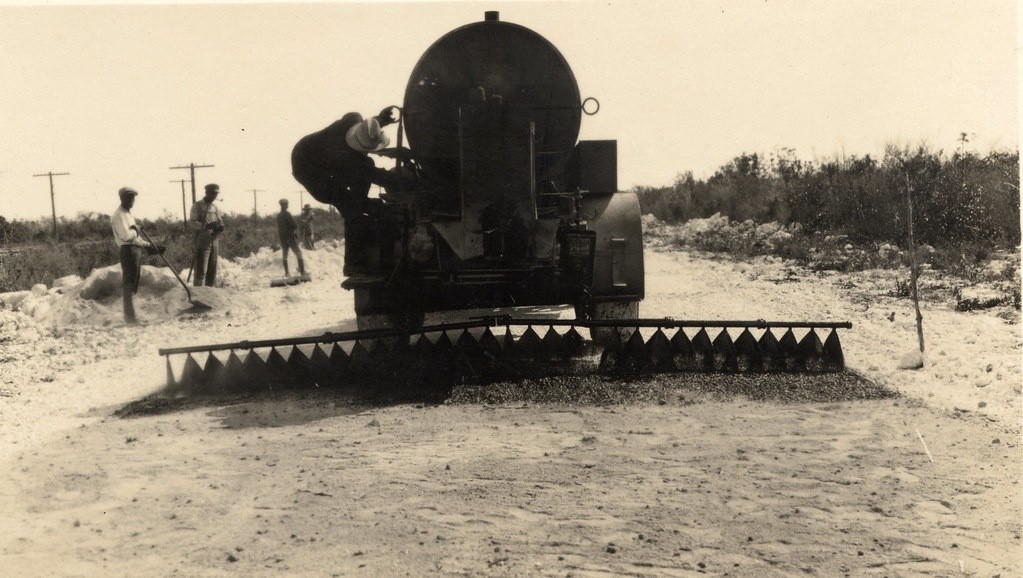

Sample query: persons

[190,184,225,288]
[276,200,309,278]
[291,111,399,278]
[109,189,151,327]
[297,204,316,250]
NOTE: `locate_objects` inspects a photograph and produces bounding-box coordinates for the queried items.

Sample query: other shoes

[344,259,384,276]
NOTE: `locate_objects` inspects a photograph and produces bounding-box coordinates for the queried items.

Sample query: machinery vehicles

[157,10,853,398]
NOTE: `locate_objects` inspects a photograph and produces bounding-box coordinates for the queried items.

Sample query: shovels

[139,226,214,311]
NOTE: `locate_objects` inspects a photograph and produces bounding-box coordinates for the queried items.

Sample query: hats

[118,187,138,196]
[204,184,221,193]
[302,204,312,210]
[346,118,390,153]
[279,199,288,205]
